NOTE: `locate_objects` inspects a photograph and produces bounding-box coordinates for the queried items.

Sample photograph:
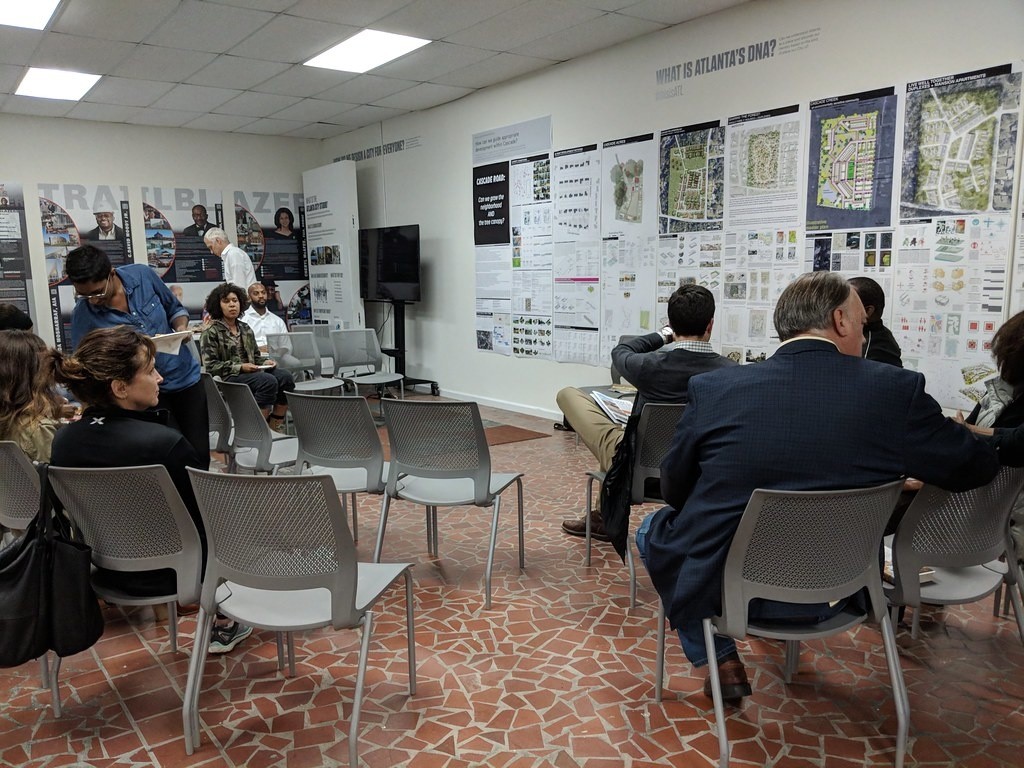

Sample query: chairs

[881,446,1024,655]
[265,324,403,410]
[201,372,296,474]
[284,390,433,562]
[182,465,415,766]
[585,402,692,606]
[213,379,309,477]
[0,440,70,685]
[373,398,521,611]
[656,473,910,767]
[34,460,287,718]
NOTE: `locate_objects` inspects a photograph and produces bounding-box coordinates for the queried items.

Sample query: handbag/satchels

[0,463,105,669]
[600,414,640,565]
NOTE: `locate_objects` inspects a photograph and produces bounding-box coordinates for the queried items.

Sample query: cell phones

[258,365,274,369]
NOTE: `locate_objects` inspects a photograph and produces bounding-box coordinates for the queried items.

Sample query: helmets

[93,200,114,215]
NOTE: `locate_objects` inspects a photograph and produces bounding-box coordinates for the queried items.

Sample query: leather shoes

[562,508,611,542]
[705,659,752,700]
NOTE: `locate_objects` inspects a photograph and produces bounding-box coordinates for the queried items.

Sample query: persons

[198,283,296,434]
[41,327,254,654]
[260,278,311,324]
[875,308,1024,581]
[86,201,123,240]
[847,276,904,369]
[181,204,219,238]
[204,227,258,293]
[272,206,298,239]
[0,300,35,332]
[0,329,73,535]
[40,199,55,214]
[143,204,155,220]
[169,285,183,302]
[0,197,8,207]
[64,246,211,471]
[635,270,1001,701]
[556,284,741,543]
[240,282,294,371]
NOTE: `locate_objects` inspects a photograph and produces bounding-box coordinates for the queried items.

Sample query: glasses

[75,272,110,300]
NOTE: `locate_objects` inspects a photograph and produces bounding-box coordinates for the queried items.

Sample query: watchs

[660,324,674,343]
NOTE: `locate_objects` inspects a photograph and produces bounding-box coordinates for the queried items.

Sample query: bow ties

[197,226,204,231]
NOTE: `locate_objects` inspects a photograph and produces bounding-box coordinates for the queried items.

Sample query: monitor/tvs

[358,224,421,302]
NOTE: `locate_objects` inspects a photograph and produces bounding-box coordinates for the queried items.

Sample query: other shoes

[267,413,286,434]
[176,602,200,616]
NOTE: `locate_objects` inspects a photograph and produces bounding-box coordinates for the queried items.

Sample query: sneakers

[207,616,253,653]
